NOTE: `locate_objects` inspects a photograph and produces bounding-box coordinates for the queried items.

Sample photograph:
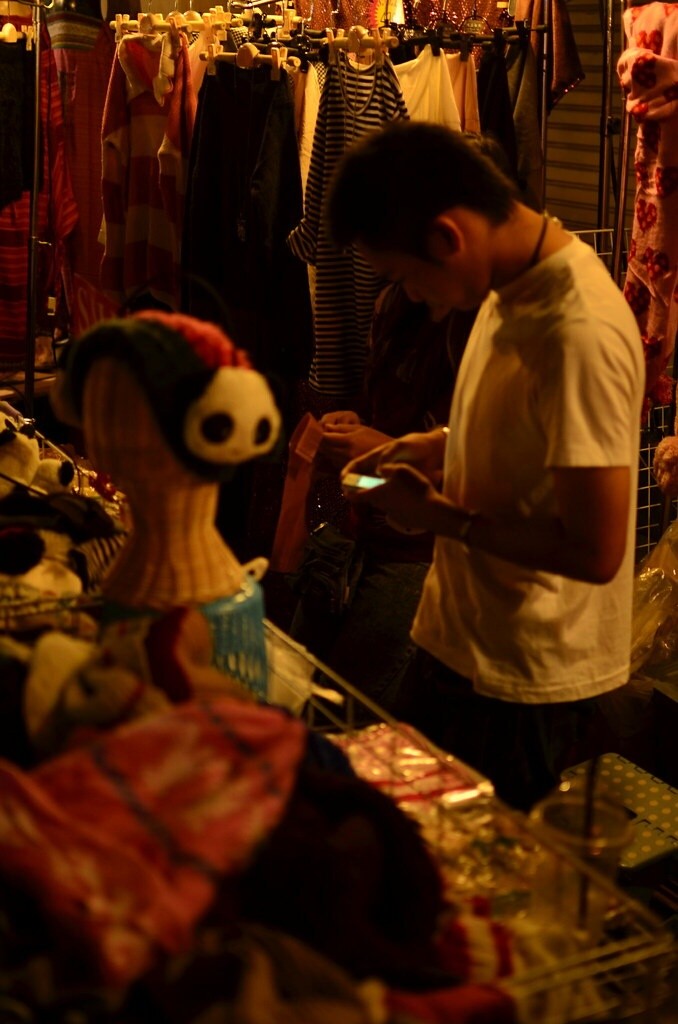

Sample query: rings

[440,426,447,432]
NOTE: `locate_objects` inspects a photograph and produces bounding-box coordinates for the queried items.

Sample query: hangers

[107,0,547,83]
[0,0,35,51]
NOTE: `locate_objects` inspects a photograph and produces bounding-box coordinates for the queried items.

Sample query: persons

[318,133,538,696]
[325,125,645,785]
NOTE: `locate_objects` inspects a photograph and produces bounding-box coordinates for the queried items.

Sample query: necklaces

[530,212,547,266]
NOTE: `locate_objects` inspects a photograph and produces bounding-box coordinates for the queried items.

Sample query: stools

[561,752,678,905]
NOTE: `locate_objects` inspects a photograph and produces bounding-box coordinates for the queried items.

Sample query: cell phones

[342,474,389,491]
